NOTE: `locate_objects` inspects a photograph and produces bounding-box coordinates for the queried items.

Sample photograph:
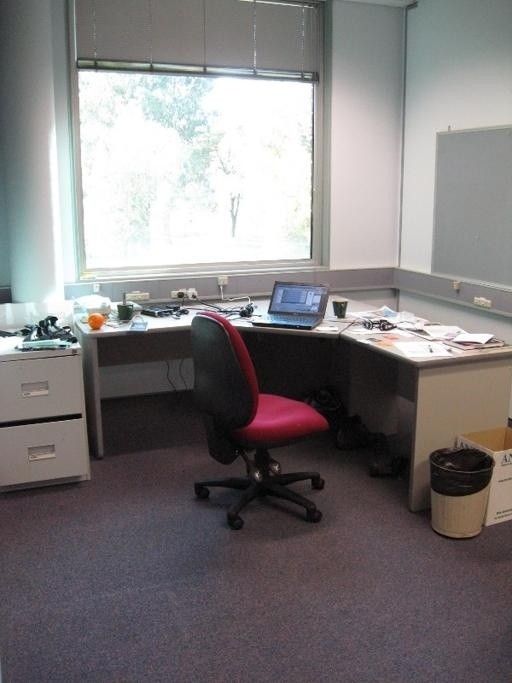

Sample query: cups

[332,301,348,318]
[118,304,134,320]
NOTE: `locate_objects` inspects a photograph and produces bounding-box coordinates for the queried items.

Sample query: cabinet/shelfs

[0,322,92,493]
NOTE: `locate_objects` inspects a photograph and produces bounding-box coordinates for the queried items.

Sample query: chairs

[189,311,330,530]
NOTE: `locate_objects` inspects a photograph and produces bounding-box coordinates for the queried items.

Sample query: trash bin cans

[428,444,495,542]
[428,344,433,353]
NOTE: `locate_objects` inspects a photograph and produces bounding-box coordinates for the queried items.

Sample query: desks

[73,298,512,512]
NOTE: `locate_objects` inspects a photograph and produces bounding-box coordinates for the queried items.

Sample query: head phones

[227,306,253,317]
[361,319,397,331]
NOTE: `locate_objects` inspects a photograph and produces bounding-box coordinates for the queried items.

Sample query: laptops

[251,281,330,331]
